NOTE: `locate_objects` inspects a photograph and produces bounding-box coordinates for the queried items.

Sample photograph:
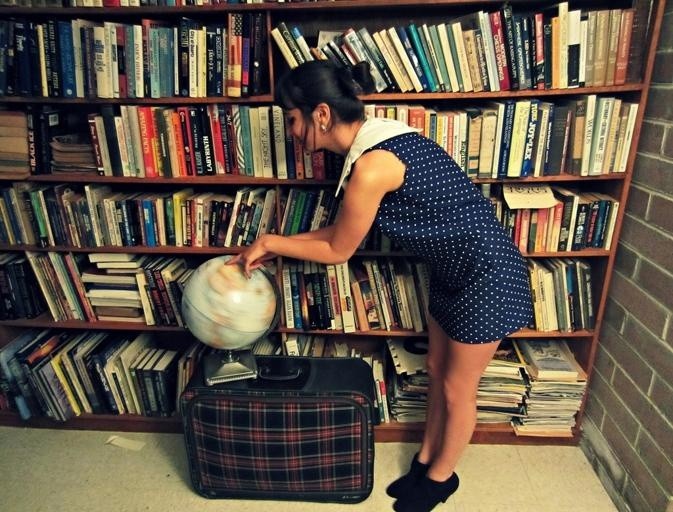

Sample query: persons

[218,59,534,512]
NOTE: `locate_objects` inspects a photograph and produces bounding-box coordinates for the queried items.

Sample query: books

[469,95,639,178]
[476,338,589,439]
[359,101,467,172]
[286,334,430,425]
[2,0,263,6]
[0,248,198,333]
[0,178,276,251]
[283,257,432,336]
[525,256,599,335]
[1,8,270,103]
[481,184,621,257]
[1,97,273,181]
[272,105,337,184]
[279,185,398,254]
[0,328,284,420]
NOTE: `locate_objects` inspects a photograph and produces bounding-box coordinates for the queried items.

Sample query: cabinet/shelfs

[0,0,668,446]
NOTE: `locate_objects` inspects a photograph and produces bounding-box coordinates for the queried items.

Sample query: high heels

[387,451,433,500]
[393,472,460,512]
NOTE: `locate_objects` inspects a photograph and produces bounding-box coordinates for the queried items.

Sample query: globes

[182,256,282,387]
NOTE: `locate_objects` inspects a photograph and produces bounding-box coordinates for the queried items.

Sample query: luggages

[179,355,377,504]
